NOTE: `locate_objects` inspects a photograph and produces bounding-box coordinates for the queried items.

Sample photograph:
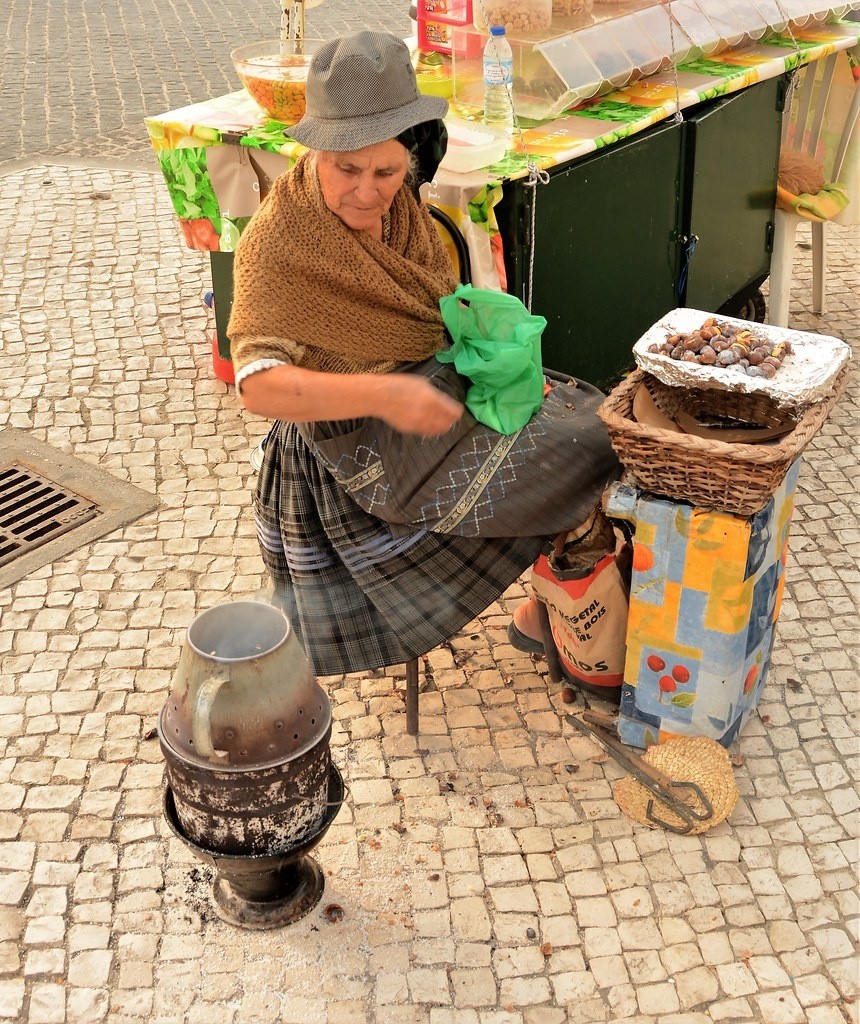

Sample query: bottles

[483,30,513,134]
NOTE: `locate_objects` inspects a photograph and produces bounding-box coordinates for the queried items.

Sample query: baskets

[595,365,849,516]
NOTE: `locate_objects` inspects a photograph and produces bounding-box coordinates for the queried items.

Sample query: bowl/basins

[230,38,326,125]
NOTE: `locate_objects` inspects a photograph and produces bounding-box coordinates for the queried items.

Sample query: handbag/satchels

[435,285,548,436]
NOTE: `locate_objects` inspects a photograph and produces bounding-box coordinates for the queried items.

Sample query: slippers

[507,620,546,655]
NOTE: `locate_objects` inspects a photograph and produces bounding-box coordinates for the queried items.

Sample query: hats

[282,30,451,151]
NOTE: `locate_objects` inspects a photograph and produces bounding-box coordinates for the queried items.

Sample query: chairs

[766,49,860,328]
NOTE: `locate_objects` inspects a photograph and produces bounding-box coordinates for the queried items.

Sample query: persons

[227,31,626,677]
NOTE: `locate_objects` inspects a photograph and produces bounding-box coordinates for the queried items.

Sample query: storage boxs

[439,0,860,174]
